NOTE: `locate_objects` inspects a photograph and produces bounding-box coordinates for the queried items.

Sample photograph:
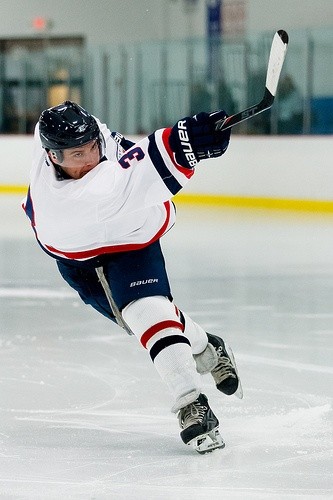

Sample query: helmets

[36,101,96,151]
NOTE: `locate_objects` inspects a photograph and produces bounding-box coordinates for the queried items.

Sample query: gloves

[167,107,235,167]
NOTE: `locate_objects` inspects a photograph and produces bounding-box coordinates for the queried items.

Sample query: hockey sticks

[213,29,289,132]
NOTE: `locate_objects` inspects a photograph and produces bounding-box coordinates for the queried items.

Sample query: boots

[175,391,226,454]
[191,328,246,399]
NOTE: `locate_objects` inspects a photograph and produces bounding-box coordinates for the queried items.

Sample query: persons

[21,99,238,444]
[189,67,305,134]
